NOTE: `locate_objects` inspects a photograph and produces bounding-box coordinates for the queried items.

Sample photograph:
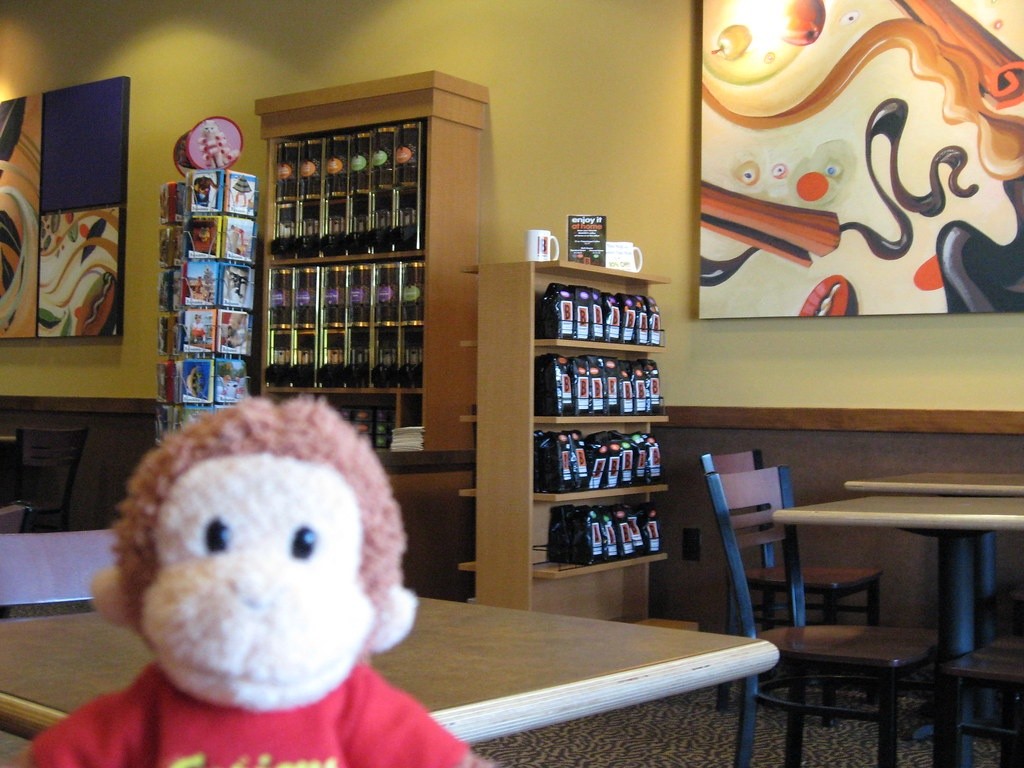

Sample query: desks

[0,597,780,746]
[842,472,1023,743]
[771,494,1024,768]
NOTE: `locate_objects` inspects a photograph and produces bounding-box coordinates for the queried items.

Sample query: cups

[522,229,559,261]
[605,241,643,273]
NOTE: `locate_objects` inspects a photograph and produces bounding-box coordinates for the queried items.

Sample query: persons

[156,174,253,434]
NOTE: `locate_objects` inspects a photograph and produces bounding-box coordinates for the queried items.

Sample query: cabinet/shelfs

[157,168,261,444]
[255,69,488,451]
[457,257,671,619]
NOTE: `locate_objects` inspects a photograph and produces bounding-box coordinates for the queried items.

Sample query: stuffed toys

[13,395,496,767]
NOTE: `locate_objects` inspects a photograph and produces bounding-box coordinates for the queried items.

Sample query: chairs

[14,426,88,533]
[940,582,1024,768]
[701,448,885,730]
[0,505,120,618]
[704,465,939,768]
